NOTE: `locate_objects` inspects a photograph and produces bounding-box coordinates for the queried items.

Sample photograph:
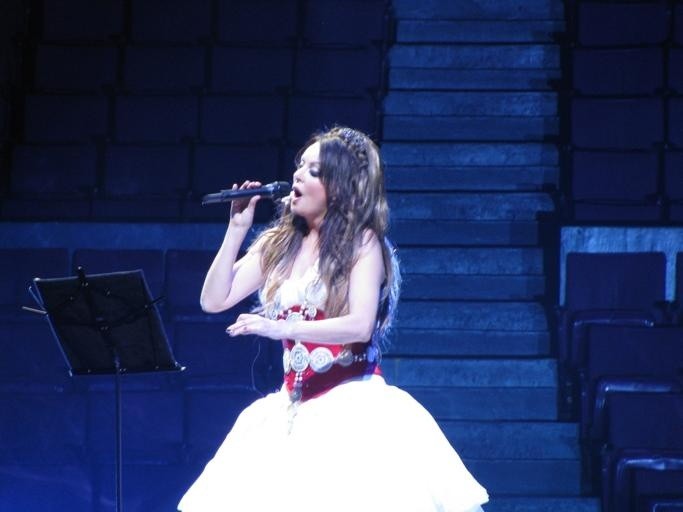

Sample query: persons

[177,126,490,512]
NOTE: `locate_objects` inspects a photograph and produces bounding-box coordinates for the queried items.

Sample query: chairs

[1,0,395,512]
[544,0,683,511]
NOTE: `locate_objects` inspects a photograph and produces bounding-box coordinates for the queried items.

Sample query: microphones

[202,181,291,205]
